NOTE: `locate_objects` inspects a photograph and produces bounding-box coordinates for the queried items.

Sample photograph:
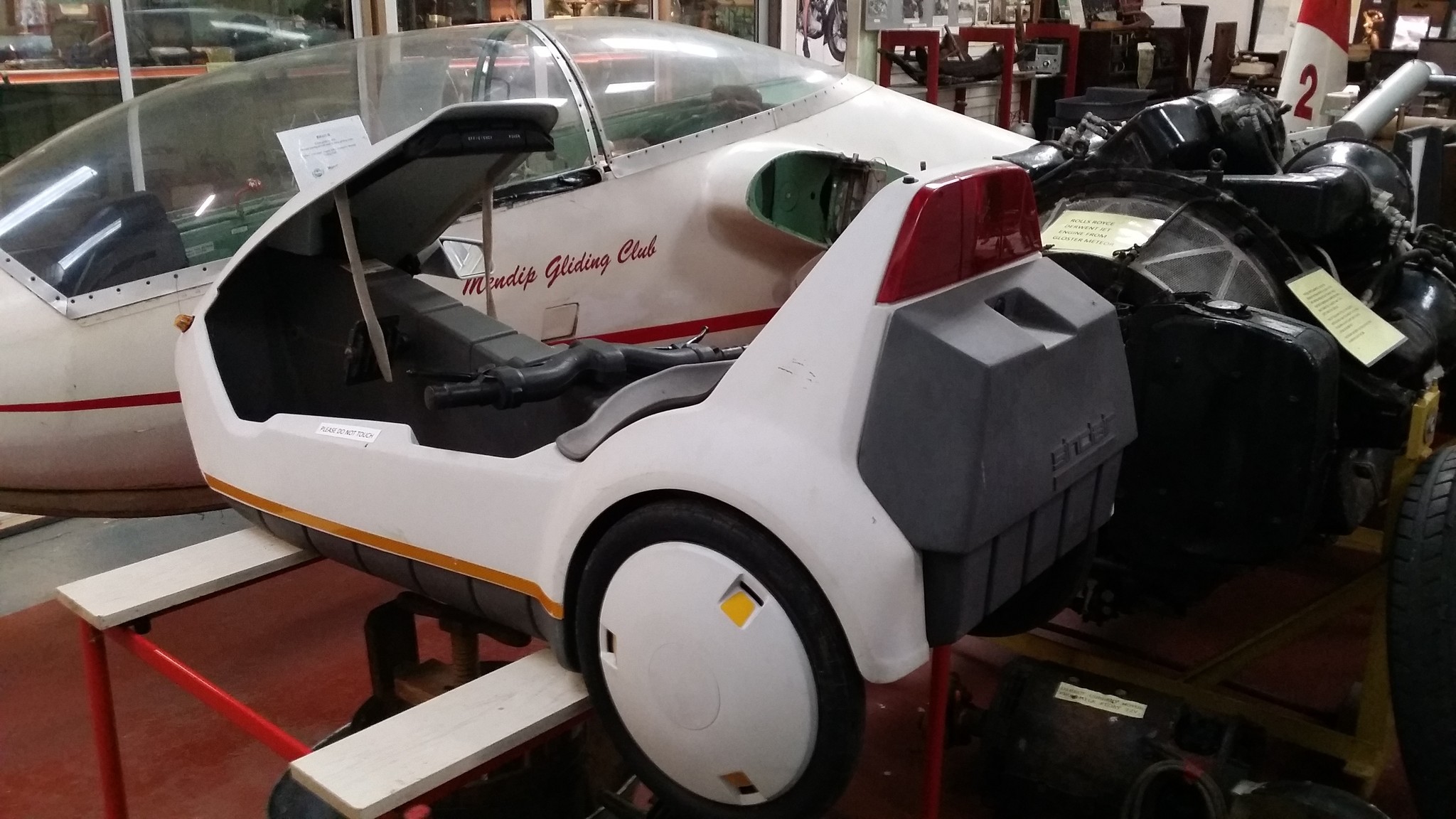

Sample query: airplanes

[1,0,1455,818]
